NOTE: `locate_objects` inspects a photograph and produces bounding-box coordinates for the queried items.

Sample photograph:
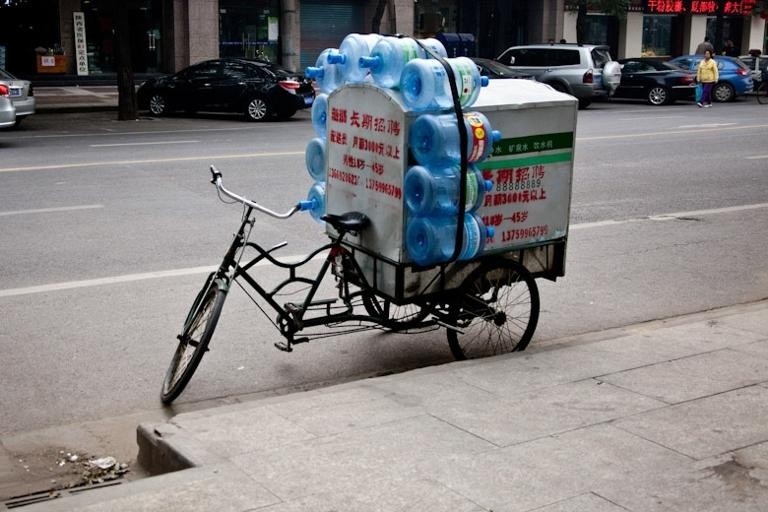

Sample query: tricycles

[160,77,578,406]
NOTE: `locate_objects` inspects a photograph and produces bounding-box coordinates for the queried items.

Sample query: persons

[696,36,715,57]
[722,36,734,57]
[696,50,720,108]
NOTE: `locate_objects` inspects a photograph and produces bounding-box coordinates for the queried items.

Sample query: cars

[138,59,315,123]
[470,57,538,89]
[609,54,768,106]
[0,67,37,128]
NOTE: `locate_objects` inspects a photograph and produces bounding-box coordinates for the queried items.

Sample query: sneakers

[696,102,713,108]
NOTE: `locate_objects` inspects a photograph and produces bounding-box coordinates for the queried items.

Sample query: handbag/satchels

[695,83,704,103]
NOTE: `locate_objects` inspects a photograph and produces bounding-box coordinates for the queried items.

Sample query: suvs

[496,40,622,112]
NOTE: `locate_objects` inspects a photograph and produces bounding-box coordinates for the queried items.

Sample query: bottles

[297,31,495,264]
